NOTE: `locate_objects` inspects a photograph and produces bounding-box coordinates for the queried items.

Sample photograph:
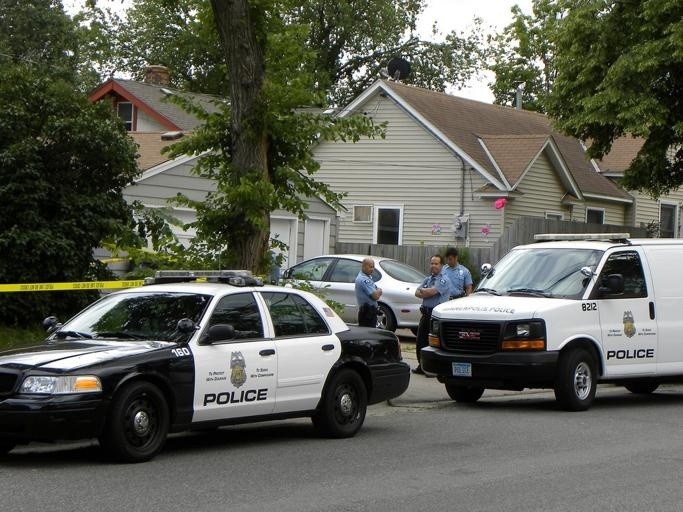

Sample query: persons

[411,255,451,374]
[441,247,474,300]
[355,259,382,328]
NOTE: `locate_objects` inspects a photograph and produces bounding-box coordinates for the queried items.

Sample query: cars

[278,253,428,336]
[0,270,410,462]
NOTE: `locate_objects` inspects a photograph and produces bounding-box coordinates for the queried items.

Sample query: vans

[420,232,683,411]
